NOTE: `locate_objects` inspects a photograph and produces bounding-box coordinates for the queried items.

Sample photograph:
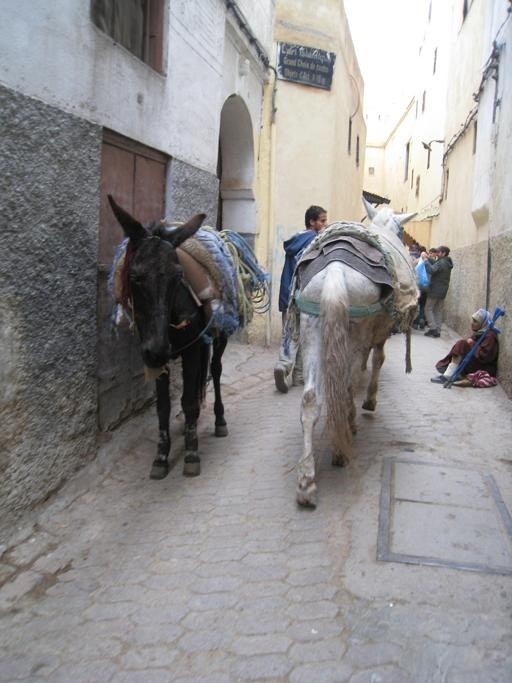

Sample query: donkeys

[106,191,241,482]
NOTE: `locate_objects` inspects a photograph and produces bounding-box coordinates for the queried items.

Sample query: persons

[410,245,453,338]
[430,309,498,384]
[273,205,328,394]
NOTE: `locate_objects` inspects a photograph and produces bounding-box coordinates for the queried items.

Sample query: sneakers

[274,363,304,393]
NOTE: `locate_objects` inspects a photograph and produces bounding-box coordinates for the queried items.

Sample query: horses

[294,195,417,510]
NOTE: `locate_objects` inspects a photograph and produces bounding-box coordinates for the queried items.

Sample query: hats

[436,246,449,252]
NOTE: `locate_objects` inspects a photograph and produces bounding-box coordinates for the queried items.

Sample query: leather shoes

[431,376,447,383]
[424,327,440,337]
